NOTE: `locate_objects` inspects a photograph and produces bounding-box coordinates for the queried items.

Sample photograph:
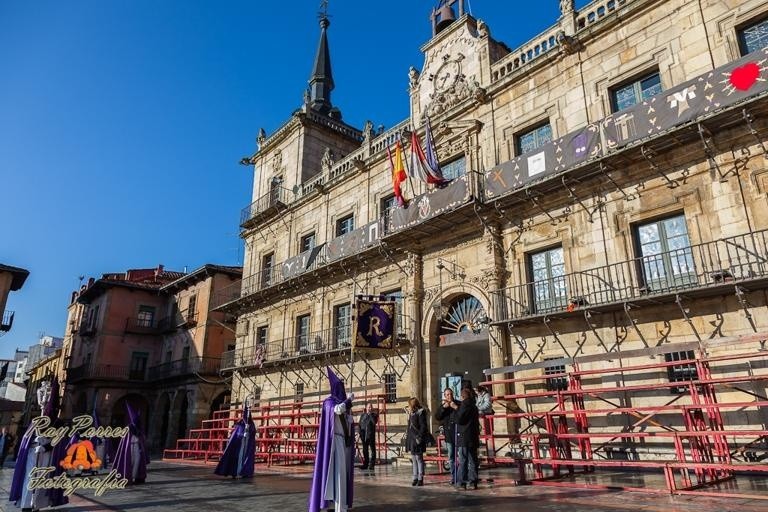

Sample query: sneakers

[412,479,424,486]
[448,479,477,491]
[359,465,375,470]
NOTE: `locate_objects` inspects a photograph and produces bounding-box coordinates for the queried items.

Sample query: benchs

[424,351,767,499]
[163,395,388,468]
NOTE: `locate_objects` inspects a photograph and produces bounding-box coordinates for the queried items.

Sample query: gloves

[34,446,44,454]
[245,424,249,430]
[346,392,354,401]
[347,415,353,424]
[34,436,45,443]
[244,433,247,437]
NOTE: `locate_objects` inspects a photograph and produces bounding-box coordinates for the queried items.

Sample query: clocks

[432,59,462,93]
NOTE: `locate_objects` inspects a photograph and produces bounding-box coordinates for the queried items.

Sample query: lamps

[433,257,465,321]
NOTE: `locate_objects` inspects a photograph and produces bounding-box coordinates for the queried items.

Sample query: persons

[474,385,493,415]
[453,389,484,490]
[434,388,463,485]
[1,401,152,510]
[357,402,379,470]
[404,398,430,486]
[213,408,257,480]
[307,382,356,511]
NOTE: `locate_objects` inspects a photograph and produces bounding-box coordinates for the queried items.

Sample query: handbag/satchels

[422,433,434,442]
[481,409,494,418]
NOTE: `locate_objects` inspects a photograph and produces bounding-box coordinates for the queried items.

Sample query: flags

[408,130,453,184]
[386,147,405,207]
[392,135,409,193]
[351,299,398,354]
[426,118,448,189]
